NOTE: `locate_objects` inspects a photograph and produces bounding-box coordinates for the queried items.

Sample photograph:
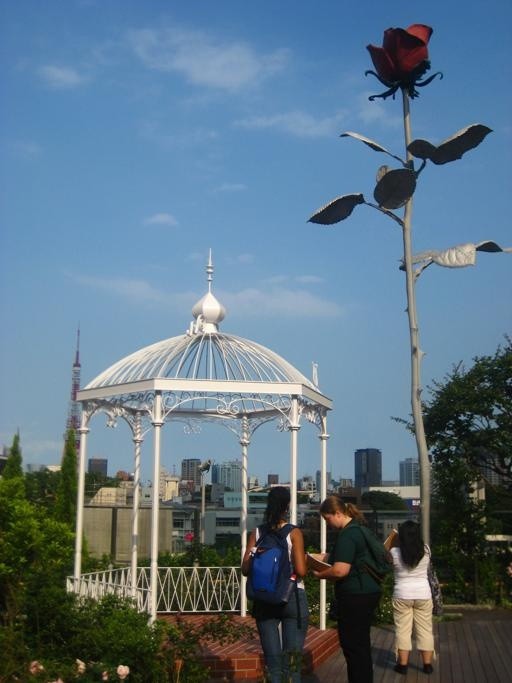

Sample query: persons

[312,496,382,683]
[241,487,308,683]
[385,520,435,674]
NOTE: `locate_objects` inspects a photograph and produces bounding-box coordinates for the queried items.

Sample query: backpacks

[247,533,290,604]
[348,523,390,584]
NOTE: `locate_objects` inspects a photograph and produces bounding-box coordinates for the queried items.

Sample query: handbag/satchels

[428,568,444,616]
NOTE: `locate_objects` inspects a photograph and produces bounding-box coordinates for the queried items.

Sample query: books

[384,529,399,552]
[306,551,332,573]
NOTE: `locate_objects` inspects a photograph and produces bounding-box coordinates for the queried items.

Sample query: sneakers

[393,664,408,674]
[422,663,434,674]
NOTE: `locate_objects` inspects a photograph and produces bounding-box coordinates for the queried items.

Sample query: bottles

[281,574,297,602]
